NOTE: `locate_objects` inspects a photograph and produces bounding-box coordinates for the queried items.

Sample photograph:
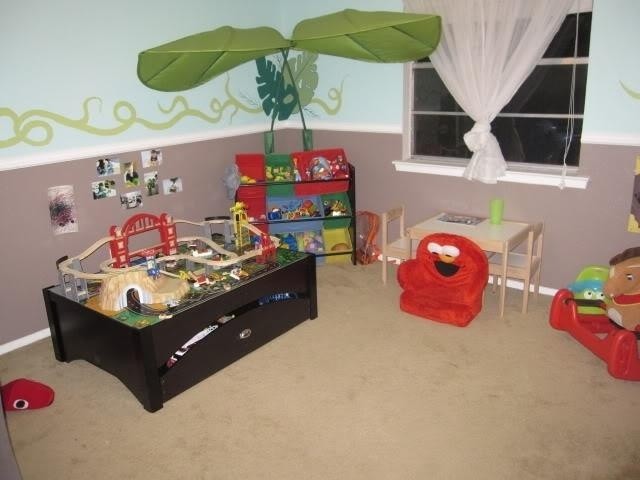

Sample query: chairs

[379,206,419,287]
[488,223,545,313]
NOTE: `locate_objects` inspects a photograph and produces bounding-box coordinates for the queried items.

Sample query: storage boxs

[270,234,303,255]
[235,153,267,201]
[323,192,354,229]
[323,229,354,265]
[291,148,350,197]
[266,151,294,199]
[237,201,267,232]
[267,199,324,234]
[302,230,325,266]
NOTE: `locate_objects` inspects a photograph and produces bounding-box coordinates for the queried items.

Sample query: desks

[403,212,532,318]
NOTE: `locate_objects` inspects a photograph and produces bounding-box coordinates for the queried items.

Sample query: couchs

[398,233,488,326]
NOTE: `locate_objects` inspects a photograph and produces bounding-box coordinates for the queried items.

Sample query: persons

[149,149,159,168]
[125,162,139,182]
[98,159,113,174]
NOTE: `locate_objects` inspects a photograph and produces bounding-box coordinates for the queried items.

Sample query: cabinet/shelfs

[41,232,319,412]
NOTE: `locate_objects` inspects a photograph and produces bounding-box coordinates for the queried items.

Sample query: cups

[489,198,504,224]
